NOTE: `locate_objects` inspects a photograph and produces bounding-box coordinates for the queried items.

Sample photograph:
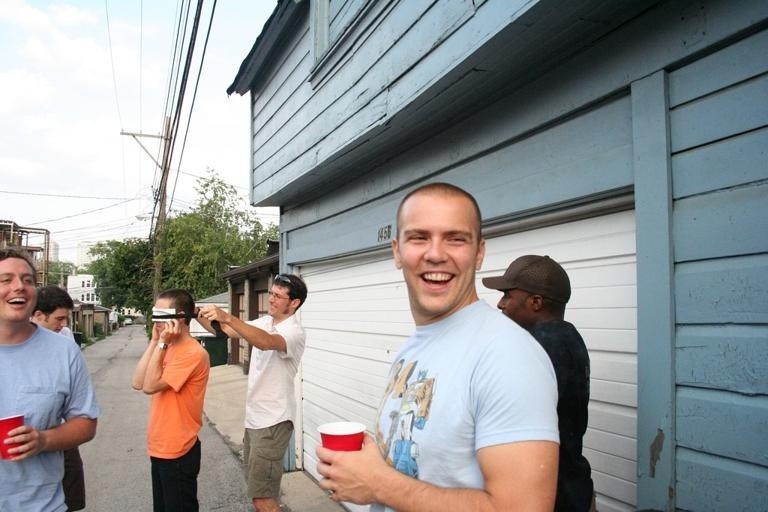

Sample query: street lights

[134,212,165,303]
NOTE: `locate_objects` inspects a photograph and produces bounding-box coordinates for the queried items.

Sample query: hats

[482,255,571,303]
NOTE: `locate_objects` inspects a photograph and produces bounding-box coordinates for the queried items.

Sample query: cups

[0,414,26,462]
[317,421,366,493]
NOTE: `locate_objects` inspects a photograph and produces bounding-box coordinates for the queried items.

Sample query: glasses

[275,274,295,286]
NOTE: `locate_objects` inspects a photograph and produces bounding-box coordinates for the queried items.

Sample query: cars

[123,317,133,325]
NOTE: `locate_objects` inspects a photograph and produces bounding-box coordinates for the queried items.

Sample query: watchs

[156,343,168,352]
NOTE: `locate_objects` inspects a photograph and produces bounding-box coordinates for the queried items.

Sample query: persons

[480,254,598,512]
[28,285,85,512]
[109,309,122,331]
[314,182,562,511]
[0,249,98,512]
[130,289,211,512]
[194,272,309,512]
